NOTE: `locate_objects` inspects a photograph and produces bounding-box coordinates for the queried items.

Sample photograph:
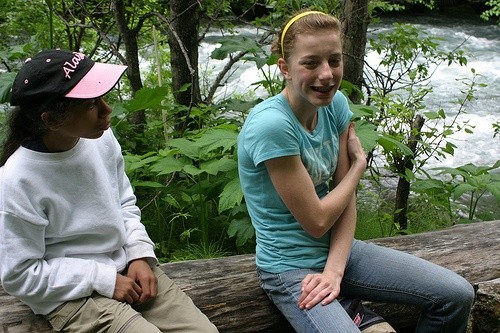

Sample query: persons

[0,48,221,333]
[237,7,476,332]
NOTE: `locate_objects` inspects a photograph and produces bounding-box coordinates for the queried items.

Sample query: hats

[9,50,128,107]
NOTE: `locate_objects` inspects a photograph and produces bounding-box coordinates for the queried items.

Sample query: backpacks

[339,297,397,333]
